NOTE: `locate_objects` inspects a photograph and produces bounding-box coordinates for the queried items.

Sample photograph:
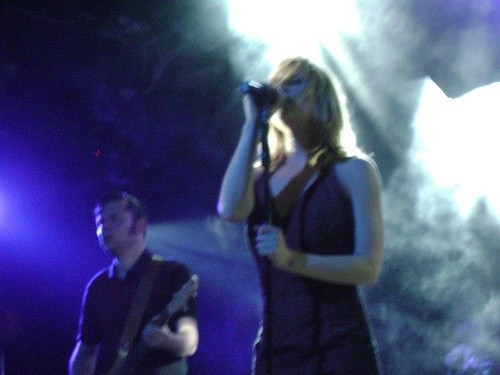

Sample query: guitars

[115,275,199,374]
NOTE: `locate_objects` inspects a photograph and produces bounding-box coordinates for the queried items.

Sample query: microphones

[242,80,287,109]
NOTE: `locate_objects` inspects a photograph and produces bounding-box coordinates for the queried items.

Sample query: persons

[69,191,199,374]
[218,55,384,375]
